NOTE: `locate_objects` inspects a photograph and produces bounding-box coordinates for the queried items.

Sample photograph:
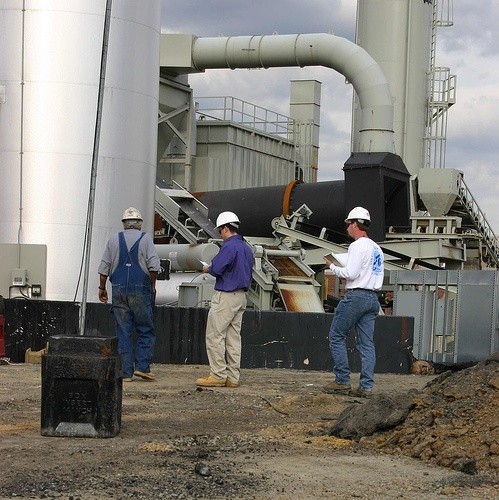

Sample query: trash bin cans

[40,334,123,438]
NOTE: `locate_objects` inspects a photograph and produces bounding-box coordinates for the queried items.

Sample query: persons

[98,206,160,382]
[323,206,384,399]
[196,211,255,387]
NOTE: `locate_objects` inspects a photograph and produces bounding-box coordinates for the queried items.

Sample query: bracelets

[98,286,106,290]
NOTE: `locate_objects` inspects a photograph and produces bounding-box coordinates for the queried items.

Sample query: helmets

[344,207,371,224]
[212,212,240,230]
[120,207,144,221]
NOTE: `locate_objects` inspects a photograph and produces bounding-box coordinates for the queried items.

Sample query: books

[323,252,348,268]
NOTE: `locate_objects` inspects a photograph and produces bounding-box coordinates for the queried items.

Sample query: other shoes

[124,377,133,382]
[349,388,372,399]
[134,370,155,380]
[196,375,226,386]
[226,379,238,387]
[323,380,351,394]
